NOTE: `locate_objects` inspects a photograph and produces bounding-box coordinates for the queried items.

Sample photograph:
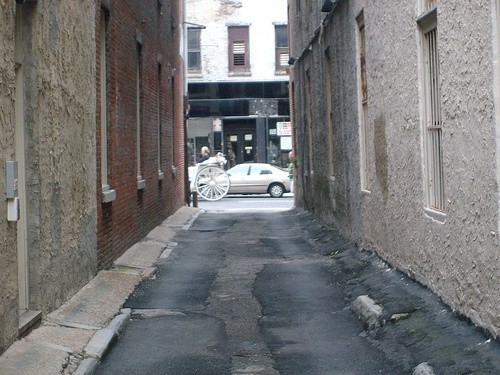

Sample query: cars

[198,163,290,196]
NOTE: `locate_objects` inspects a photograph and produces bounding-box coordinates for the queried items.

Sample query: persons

[288,151,295,194]
[198,146,212,163]
[216,152,226,171]
